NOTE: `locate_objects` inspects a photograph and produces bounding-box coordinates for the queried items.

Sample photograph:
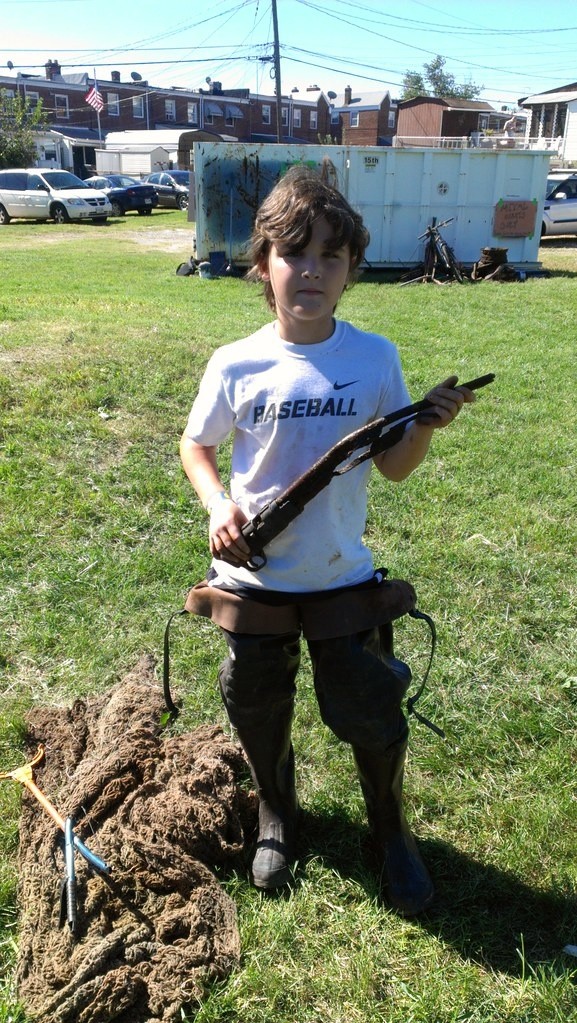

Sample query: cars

[131,170,190,211]
[83,173,159,217]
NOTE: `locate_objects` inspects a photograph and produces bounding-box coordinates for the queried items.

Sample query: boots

[238,720,311,889]
[352,728,435,916]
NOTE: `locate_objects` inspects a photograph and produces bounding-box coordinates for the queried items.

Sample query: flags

[85,87,104,112]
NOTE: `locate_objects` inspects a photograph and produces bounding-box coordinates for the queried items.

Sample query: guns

[231,371,497,573]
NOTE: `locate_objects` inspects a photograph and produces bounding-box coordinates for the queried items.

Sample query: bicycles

[417,218,464,285]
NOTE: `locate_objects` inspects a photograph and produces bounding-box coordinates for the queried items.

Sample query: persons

[179,157,475,916]
[504,116,517,141]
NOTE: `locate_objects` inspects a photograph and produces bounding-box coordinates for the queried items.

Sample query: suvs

[541,168,577,237]
[0,168,111,224]
